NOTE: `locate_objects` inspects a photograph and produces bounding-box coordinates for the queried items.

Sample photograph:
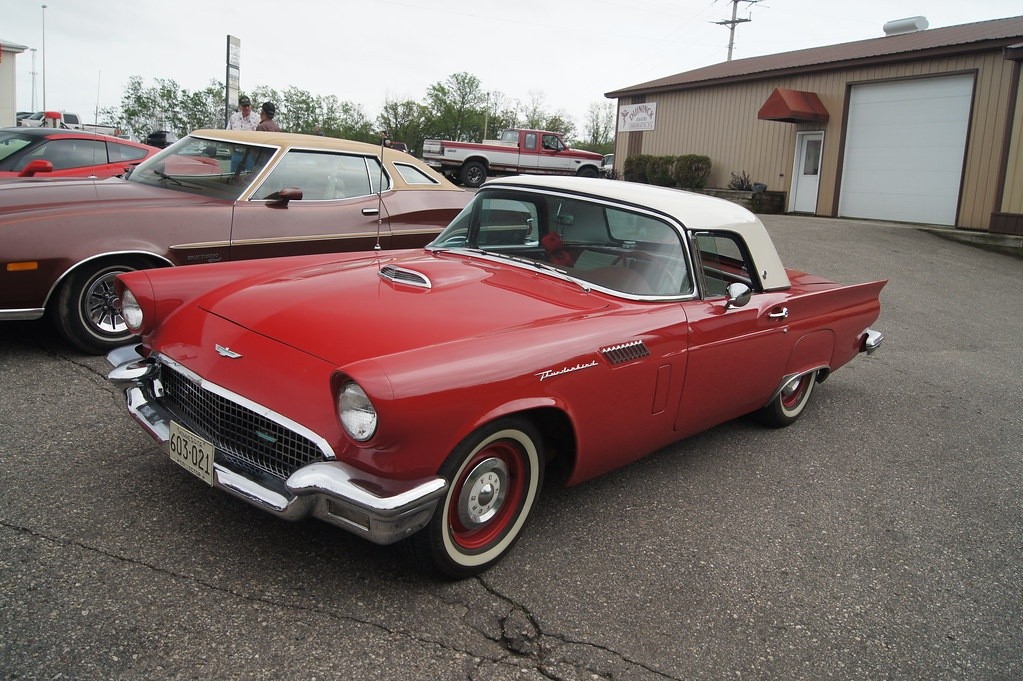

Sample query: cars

[116,135,139,143]
[0,128,220,182]
[17,112,34,125]
[105,175,890,580]
[385,142,408,155]
[186,144,206,155]
[604,154,614,172]
[217,149,232,160]
[0,129,533,354]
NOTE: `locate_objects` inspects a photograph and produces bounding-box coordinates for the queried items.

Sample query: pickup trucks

[422,127,606,188]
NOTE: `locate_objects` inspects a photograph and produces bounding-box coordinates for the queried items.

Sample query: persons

[316,127,324,135]
[381,131,392,148]
[253,102,279,166]
[225,95,262,173]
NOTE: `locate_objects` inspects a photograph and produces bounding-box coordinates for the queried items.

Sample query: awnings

[758,87,829,121]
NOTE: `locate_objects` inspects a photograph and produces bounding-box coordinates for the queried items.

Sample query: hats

[261,102,275,115]
[239,96,251,106]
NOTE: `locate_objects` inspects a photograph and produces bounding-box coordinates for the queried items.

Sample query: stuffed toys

[542,232,574,266]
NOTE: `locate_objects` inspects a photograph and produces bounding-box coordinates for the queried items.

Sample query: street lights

[42,5,47,111]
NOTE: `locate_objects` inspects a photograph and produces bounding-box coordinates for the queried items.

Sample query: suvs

[147,131,177,150]
[20,110,83,132]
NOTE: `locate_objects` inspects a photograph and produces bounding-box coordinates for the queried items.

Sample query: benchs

[573,251,752,296]
